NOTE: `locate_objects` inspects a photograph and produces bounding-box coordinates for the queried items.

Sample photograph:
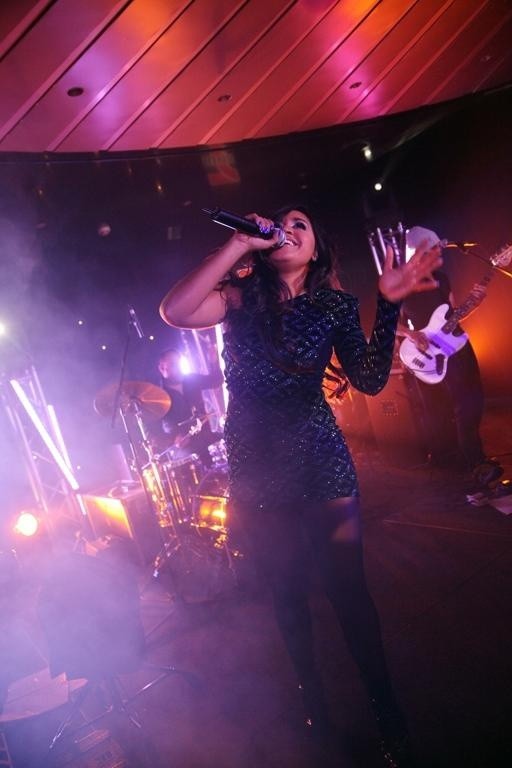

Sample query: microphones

[440,238,480,248]
[201,203,286,248]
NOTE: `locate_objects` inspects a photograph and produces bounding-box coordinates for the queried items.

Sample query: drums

[193,467,236,553]
[141,454,202,530]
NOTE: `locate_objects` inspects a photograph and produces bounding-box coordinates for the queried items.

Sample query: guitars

[398,244,512,384]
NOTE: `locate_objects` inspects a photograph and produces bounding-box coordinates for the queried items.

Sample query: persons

[396,223,510,507]
[158,202,444,768]
[156,343,222,466]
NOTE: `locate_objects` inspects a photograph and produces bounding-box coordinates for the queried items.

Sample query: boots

[358,669,413,767]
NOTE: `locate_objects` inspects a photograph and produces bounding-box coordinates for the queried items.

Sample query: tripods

[45,663,201,768]
[113,407,222,639]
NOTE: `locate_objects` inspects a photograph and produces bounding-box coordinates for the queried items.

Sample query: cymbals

[176,410,214,426]
[93,380,172,427]
